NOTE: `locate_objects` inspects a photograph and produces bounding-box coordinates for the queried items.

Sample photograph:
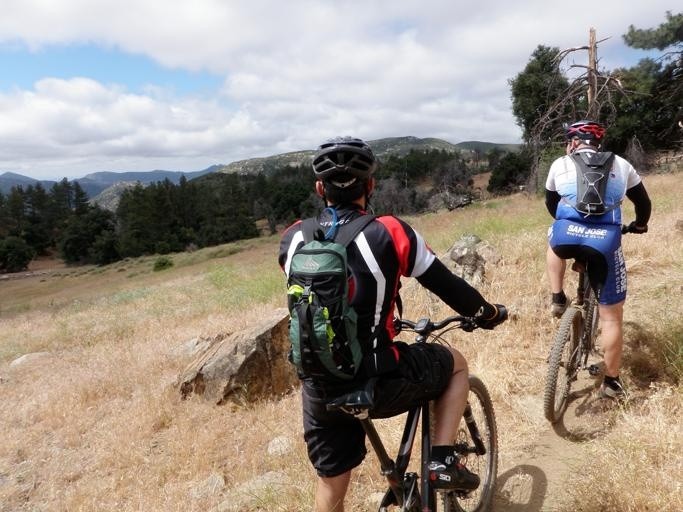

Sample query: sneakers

[551,298,568,315]
[428,456,481,492]
[602,383,622,398]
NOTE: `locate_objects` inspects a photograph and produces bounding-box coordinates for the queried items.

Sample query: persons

[278,137,507,511]
[543,119,651,399]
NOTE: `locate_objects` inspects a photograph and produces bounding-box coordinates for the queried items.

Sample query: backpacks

[286,213,375,386]
[571,151,613,217]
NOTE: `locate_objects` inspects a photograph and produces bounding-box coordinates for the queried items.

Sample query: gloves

[475,304,509,331]
[628,222,648,234]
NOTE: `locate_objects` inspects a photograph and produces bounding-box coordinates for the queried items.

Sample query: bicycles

[542,219,651,426]
[326,309,509,512]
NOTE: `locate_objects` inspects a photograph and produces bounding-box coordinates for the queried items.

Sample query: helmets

[311,136,376,183]
[566,121,606,145]
